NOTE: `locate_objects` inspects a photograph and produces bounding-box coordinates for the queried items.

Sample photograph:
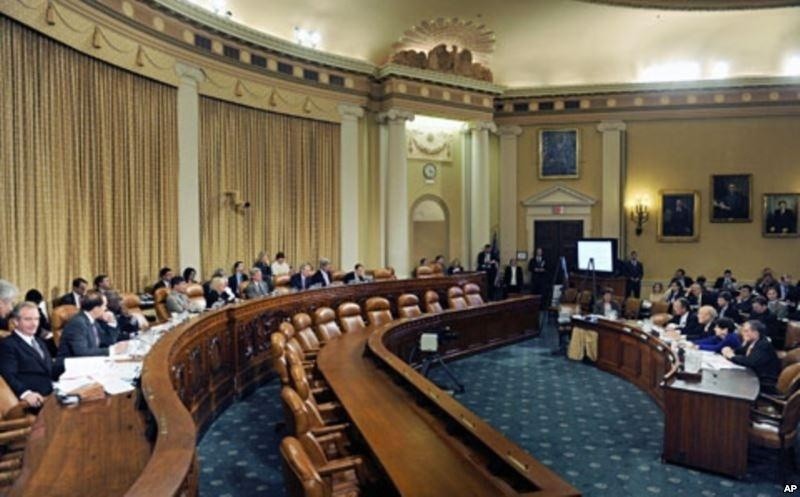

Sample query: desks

[572,307,760,480]
[3,270,488,496]
[315,292,583,497]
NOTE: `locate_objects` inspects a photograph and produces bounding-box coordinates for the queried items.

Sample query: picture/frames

[706,171,755,224]
[655,187,699,242]
[538,126,581,180]
[762,190,800,239]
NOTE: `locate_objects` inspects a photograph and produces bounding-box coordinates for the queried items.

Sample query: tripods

[421,355,466,394]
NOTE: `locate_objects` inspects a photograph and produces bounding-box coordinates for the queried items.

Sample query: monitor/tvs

[574,238,618,277]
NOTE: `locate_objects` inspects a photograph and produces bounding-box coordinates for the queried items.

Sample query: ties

[31,340,42,359]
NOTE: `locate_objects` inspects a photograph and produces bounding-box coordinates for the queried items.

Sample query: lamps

[627,193,651,236]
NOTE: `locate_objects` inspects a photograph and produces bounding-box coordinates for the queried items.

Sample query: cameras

[416,325,461,354]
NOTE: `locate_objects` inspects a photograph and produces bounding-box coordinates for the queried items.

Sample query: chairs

[749,391,799,484]
[338,302,365,332]
[313,306,342,342]
[270,331,335,423]
[278,320,320,375]
[239,281,249,300]
[424,289,443,312]
[612,294,625,307]
[118,294,150,330]
[430,263,445,277]
[648,299,674,326]
[279,385,379,489]
[397,293,421,318]
[372,266,396,281]
[623,296,643,321]
[330,271,347,283]
[579,290,596,316]
[417,266,432,278]
[292,312,328,353]
[272,274,291,294]
[463,283,484,307]
[155,288,173,322]
[285,353,365,448]
[557,288,580,346]
[365,297,394,324]
[185,283,206,311]
[0,377,37,497]
[758,362,800,412]
[448,286,468,309]
[739,301,800,364]
[279,435,378,496]
[50,304,81,350]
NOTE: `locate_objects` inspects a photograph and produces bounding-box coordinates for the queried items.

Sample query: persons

[670,199,691,234]
[685,284,717,307]
[105,291,140,339]
[252,252,274,291]
[270,253,289,278]
[664,283,686,303]
[714,291,739,322]
[344,264,365,284]
[756,268,777,293]
[61,290,131,356]
[0,281,19,337]
[668,268,694,288]
[212,270,226,286]
[721,320,781,391]
[245,267,268,298]
[478,246,498,296]
[165,276,196,316]
[770,200,795,233]
[447,258,465,274]
[733,284,756,312]
[778,272,799,303]
[504,258,524,297]
[25,290,58,356]
[759,274,781,299]
[696,277,711,294]
[667,305,722,342]
[592,287,620,320]
[207,279,230,306]
[624,251,643,298]
[433,256,447,272]
[226,261,248,290]
[664,294,697,334]
[289,263,312,291]
[63,279,89,306]
[181,267,197,285]
[0,300,60,415]
[92,275,112,295]
[154,268,173,295]
[648,282,665,325]
[765,285,788,319]
[312,258,333,288]
[413,258,429,275]
[747,296,778,336]
[528,248,548,299]
[717,184,748,218]
[714,269,736,290]
[677,317,742,354]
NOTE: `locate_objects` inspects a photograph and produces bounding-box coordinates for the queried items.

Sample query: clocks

[423,162,437,179]
